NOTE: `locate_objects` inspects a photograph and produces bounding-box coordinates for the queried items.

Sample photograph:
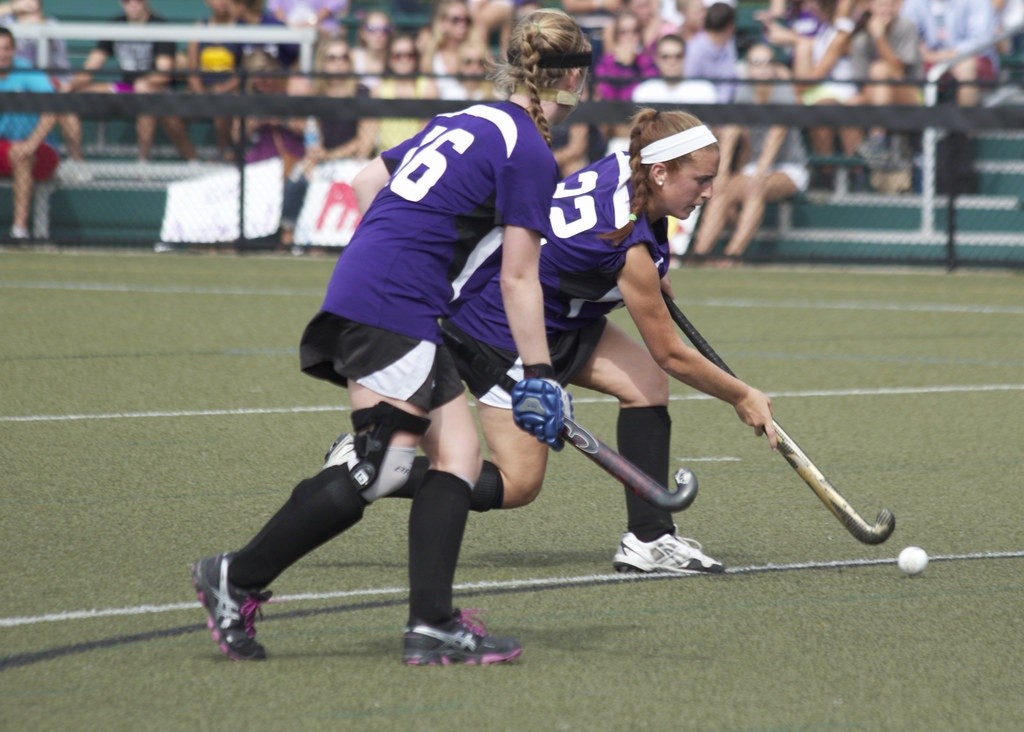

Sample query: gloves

[511,378,575,452]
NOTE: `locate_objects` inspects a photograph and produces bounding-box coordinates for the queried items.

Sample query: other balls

[896,544,929,575]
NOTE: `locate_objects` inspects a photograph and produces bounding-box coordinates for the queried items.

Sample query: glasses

[327,54,350,62]
[462,59,486,66]
[660,53,684,60]
[444,16,472,27]
[616,28,640,35]
[390,52,418,60]
[366,25,391,34]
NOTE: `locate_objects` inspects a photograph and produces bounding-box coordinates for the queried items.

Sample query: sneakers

[318,433,357,473]
[612,523,725,575]
[401,609,524,666]
[190,552,273,662]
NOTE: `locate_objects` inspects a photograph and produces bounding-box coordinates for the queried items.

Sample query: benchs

[1,0,1023,264]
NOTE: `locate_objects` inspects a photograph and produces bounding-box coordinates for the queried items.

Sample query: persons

[322,110,778,575]
[0,0,1024,270]
[192,9,593,667]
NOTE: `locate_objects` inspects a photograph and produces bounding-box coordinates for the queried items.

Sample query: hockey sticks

[437,311,702,516]
[657,279,901,547]
